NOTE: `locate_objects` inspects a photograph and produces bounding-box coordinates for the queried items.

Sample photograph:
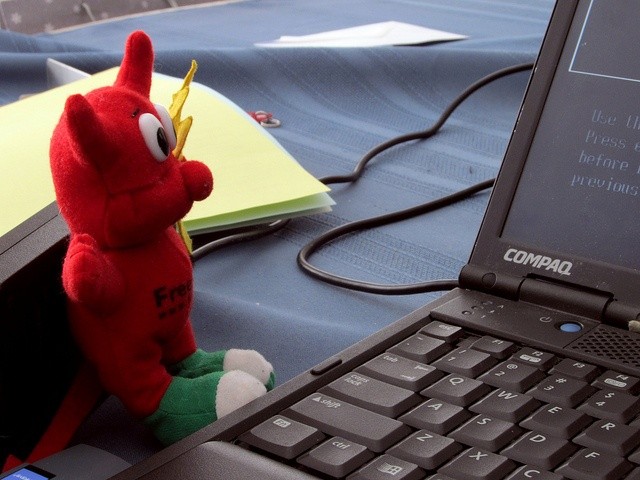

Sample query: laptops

[107,1,640,480]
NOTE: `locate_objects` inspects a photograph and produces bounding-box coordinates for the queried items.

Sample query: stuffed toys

[0,31,275,473]
